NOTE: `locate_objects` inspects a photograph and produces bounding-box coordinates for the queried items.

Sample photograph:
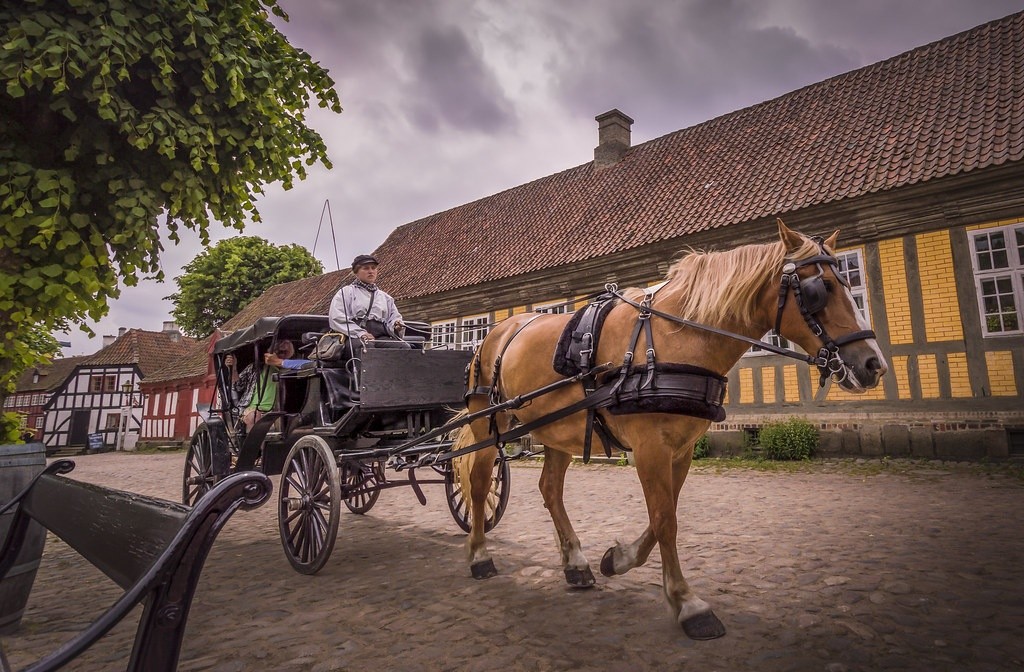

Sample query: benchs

[303,332,426,408]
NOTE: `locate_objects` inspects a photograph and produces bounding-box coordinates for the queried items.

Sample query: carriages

[181,218,888,641]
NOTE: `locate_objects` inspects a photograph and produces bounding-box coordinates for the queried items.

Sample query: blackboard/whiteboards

[88,433,104,450]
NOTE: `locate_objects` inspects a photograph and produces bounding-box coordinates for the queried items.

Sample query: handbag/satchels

[308,333,345,361]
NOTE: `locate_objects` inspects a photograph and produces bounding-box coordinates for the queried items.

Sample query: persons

[224,339,294,468]
[329,255,411,385]
[264,327,336,370]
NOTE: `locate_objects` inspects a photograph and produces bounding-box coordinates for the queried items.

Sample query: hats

[354,255,378,265]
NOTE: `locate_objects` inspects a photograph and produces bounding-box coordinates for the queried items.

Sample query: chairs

[0,458,273,672]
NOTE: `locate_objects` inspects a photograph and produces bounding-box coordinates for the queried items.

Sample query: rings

[227,360,230,362]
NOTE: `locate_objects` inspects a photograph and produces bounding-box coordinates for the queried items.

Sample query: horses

[443,216,888,640]
